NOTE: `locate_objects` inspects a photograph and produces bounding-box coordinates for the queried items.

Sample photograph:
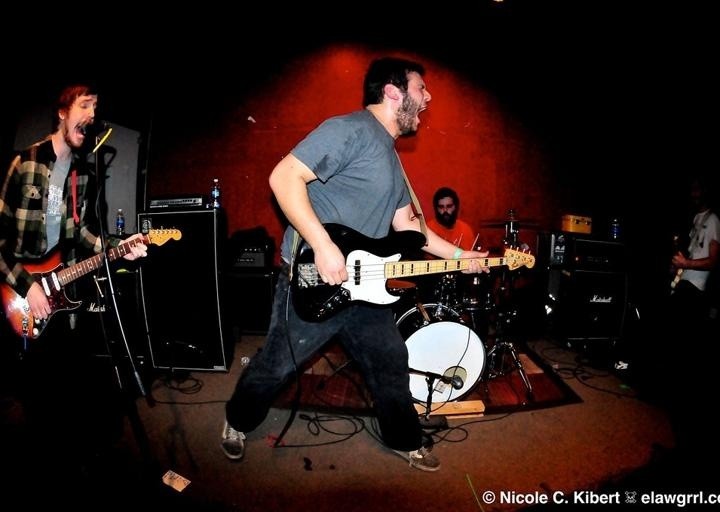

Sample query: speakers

[229,269,279,337]
[136,210,234,372]
[542,234,628,341]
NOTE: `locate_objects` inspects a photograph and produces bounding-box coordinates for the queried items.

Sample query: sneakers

[219,419,246,460]
[391,447,442,473]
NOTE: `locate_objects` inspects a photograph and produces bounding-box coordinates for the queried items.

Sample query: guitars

[288,223,536,324]
[0,226,182,346]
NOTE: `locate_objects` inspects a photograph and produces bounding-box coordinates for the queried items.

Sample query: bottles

[209,176,223,208]
[116,207,125,235]
[505,208,519,242]
[611,218,621,240]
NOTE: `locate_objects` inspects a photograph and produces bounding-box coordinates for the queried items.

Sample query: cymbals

[481,219,543,230]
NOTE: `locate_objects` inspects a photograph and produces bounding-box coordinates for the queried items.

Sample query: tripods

[486,291,535,402]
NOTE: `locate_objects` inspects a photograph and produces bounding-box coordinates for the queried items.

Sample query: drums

[461,270,495,311]
[431,270,463,313]
[396,302,486,403]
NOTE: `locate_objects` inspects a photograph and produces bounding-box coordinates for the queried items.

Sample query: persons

[424,186,474,253]
[221,56,490,472]
[670,183,719,313]
[0,85,151,362]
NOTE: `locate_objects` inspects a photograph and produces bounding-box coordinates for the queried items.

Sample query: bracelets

[451,248,463,259]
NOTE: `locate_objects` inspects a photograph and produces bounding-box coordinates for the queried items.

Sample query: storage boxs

[560,214,592,234]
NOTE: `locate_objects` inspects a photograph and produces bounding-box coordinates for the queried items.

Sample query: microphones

[80,119,113,136]
[434,375,464,390]
